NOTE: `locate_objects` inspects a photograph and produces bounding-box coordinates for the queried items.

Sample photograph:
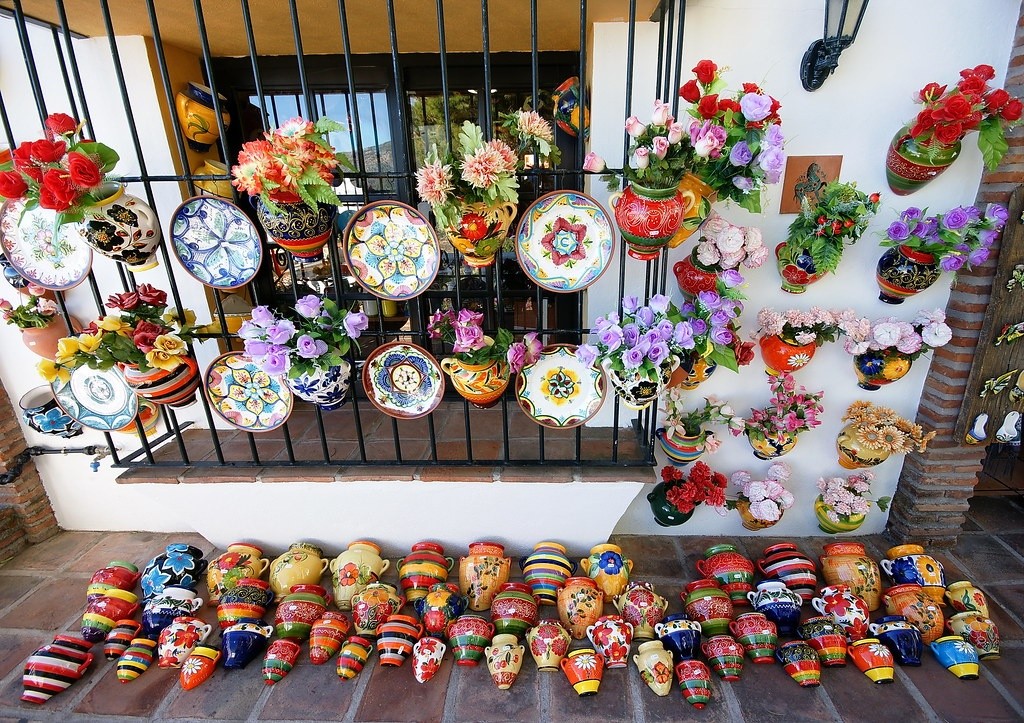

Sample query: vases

[746,427,798,460]
[74,181,160,272]
[647,479,696,527]
[118,354,202,410]
[19,385,83,439]
[446,199,518,267]
[551,77,590,142]
[20,542,1000,709]
[609,182,696,259]
[192,159,242,205]
[214,294,254,342]
[280,357,360,410]
[814,494,866,534]
[601,355,680,410]
[673,254,725,303]
[666,349,696,387]
[247,189,338,262]
[737,498,785,531]
[440,356,512,409]
[876,244,939,305]
[886,126,962,195]
[16,282,65,303]
[19,312,83,360]
[681,355,718,389]
[837,423,891,469]
[655,426,715,466]
[175,82,230,153]
[668,170,718,249]
[853,345,912,391]
[775,242,831,293]
[0,253,30,287]
[116,395,159,438]
[759,331,816,378]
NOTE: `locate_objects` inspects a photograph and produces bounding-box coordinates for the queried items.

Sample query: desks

[347,253,535,321]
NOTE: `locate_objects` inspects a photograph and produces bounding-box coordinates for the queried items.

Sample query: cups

[381,299,397,318]
[362,299,378,318]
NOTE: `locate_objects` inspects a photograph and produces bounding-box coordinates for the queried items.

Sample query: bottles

[1,251,65,304]
[175,80,231,155]
[553,76,592,140]
[21,544,1003,710]
[192,160,238,204]
[19,385,83,437]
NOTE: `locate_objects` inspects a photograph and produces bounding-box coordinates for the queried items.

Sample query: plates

[204,350,293,432]
[343,200,441,301]
[362,341,445,420]
[49,356,138,432]
[515,342,608,429]
[516,189,616,293]
[0,197,93,291]
[169,196,262,289]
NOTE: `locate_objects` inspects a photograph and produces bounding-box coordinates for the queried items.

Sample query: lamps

[800,0,869,92]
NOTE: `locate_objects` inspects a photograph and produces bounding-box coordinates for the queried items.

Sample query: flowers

[0,65,1024,523]
